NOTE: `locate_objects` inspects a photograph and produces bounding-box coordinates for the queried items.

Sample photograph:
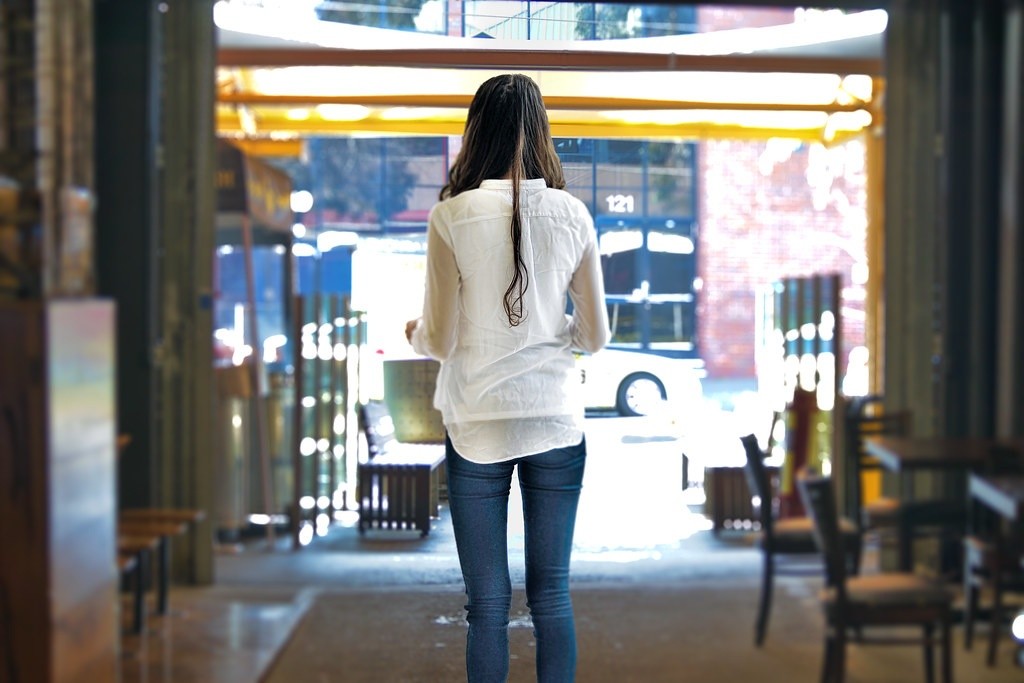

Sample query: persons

[405,74,613,683]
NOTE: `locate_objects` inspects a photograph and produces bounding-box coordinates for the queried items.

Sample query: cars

[579,343,704,419]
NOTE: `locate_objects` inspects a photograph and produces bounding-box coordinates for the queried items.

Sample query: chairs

[960,533,1023,667]
[797,467,962,683]
[736,429,863,647]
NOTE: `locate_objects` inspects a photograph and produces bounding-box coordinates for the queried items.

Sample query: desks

[863,432,1023,573]
[966,466,1023,524]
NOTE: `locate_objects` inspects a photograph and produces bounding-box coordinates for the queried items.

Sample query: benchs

[117,506,210,630]
[673,389,782,535]
[356,398,447,538]
